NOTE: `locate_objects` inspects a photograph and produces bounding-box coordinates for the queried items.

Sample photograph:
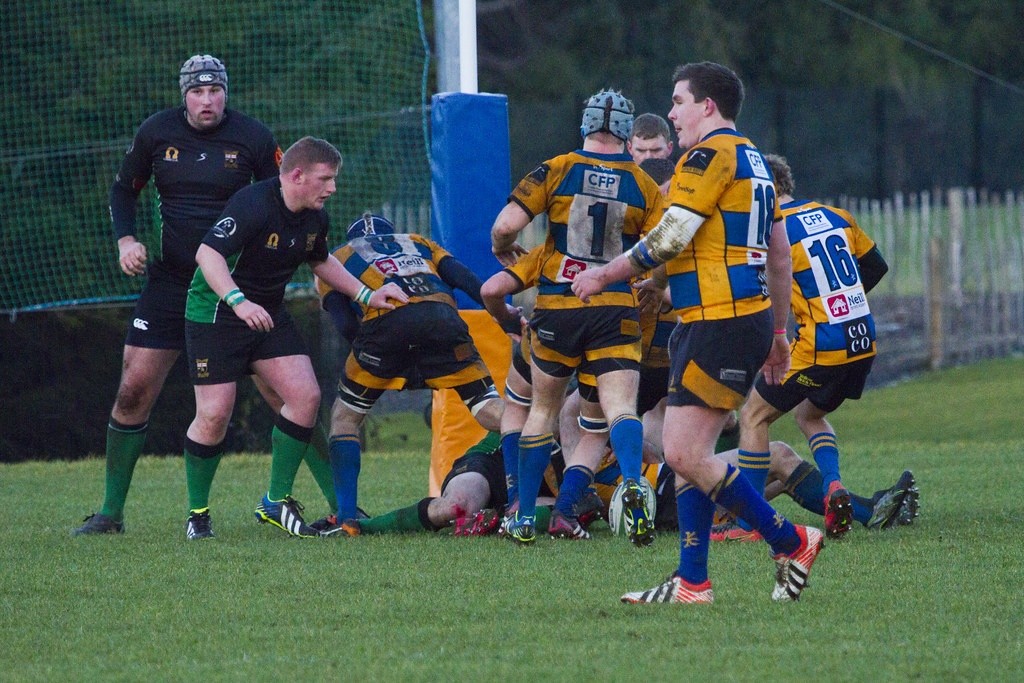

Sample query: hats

[179,54,227,107]
[581,87,633,144]
[345,214,395,241]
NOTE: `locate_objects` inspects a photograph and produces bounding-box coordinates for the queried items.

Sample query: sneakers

[70,513,125,538]
[310,514,361,538]
[186,507,215,542]
[709,515,765,542]
[772,524,825,605]
[577,492,604,529]
[897,487,919,526]
[864,471,915,532]
[255,493,319,539]
[621,570,713,605]
[549,510,592,539]
[620,478,656,547]
[505,510,536,546]
[823,480,852,540]
[448,506,498,537]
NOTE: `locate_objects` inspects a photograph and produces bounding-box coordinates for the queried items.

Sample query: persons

[568,61,825,603]
[71,53,413,544]
[320,87,921,548]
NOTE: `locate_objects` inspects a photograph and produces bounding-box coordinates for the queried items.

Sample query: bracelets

[224,289,246,307]
[773,330,787,337]
[354,285,375,307]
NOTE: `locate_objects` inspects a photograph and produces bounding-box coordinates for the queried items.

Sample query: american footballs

[609,476,657,538]
